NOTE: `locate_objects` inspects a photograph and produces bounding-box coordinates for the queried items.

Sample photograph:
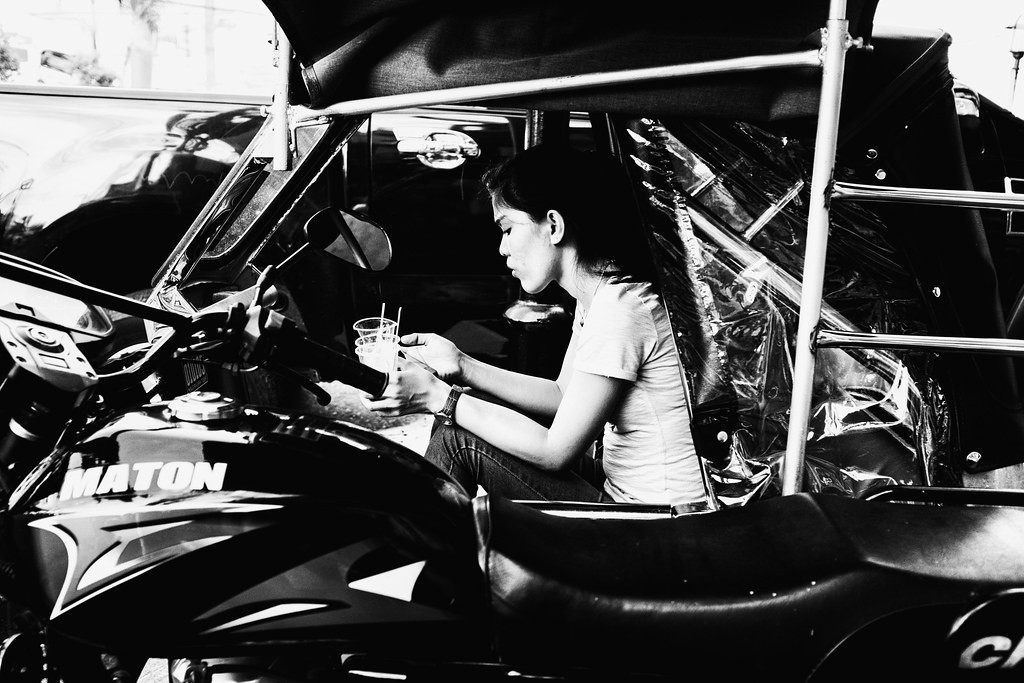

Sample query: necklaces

[580,259,615,327]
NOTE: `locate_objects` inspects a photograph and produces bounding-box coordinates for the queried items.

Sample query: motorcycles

[0,92,1024,683]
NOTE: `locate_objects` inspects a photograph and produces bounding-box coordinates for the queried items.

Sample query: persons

[359,139,716,505]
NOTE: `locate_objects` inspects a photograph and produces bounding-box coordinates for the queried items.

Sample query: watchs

[435,384,463,426]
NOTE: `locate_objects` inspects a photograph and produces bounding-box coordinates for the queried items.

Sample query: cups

[354,317,400,384]
